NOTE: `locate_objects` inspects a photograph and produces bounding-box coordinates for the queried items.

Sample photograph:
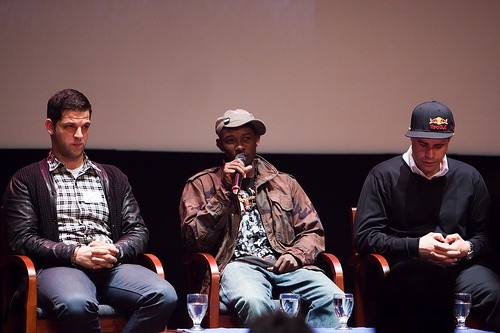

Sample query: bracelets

[72,244,85,267]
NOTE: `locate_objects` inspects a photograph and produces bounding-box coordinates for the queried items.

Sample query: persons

[179,109,345,327]
[2,88,178,333]
[353,100,500,330]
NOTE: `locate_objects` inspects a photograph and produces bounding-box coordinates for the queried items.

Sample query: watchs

[467,242,474,261]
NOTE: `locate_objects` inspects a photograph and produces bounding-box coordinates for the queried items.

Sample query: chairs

[183,237,344,328]
[0,209,165,333]
[350,207,495,333]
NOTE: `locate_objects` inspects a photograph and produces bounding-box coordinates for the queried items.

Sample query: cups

[279,292,302,317]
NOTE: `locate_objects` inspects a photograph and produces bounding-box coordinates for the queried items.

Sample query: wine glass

[454,293,473,330]
[186,294,208,330]
[333,292,354,330]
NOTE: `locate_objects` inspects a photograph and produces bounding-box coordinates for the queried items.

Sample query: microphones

[232,154,247,193]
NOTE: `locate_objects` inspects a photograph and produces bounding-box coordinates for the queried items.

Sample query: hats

[405,100,455,139]
[215,109,267,137]
